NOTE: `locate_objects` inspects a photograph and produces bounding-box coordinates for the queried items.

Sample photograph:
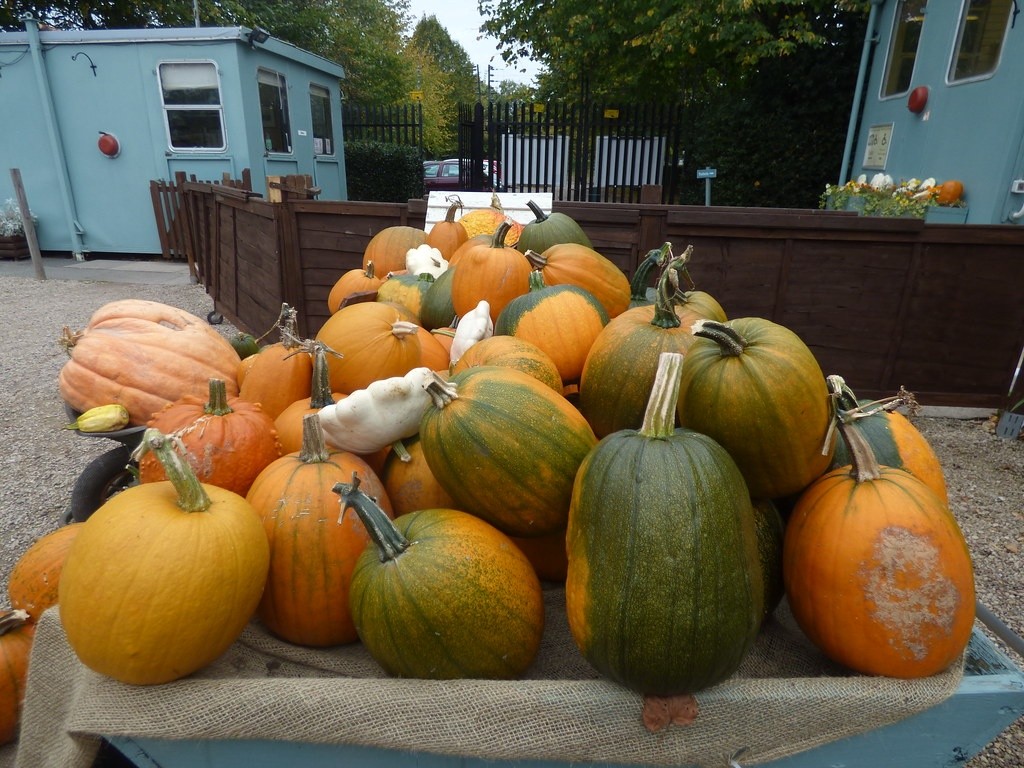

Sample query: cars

[423,158,501,195]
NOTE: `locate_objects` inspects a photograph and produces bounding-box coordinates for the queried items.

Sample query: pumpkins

[0,199,976,747]
[936,180,963,204]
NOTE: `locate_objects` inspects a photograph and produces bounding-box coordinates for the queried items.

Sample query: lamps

[245,26,271,50]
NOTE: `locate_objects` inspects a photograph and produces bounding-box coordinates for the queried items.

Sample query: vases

[825,190,969,224]
[0,236,31,262]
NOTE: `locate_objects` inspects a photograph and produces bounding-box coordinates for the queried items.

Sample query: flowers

[0,198,39,237]
[819,180,966,218]
